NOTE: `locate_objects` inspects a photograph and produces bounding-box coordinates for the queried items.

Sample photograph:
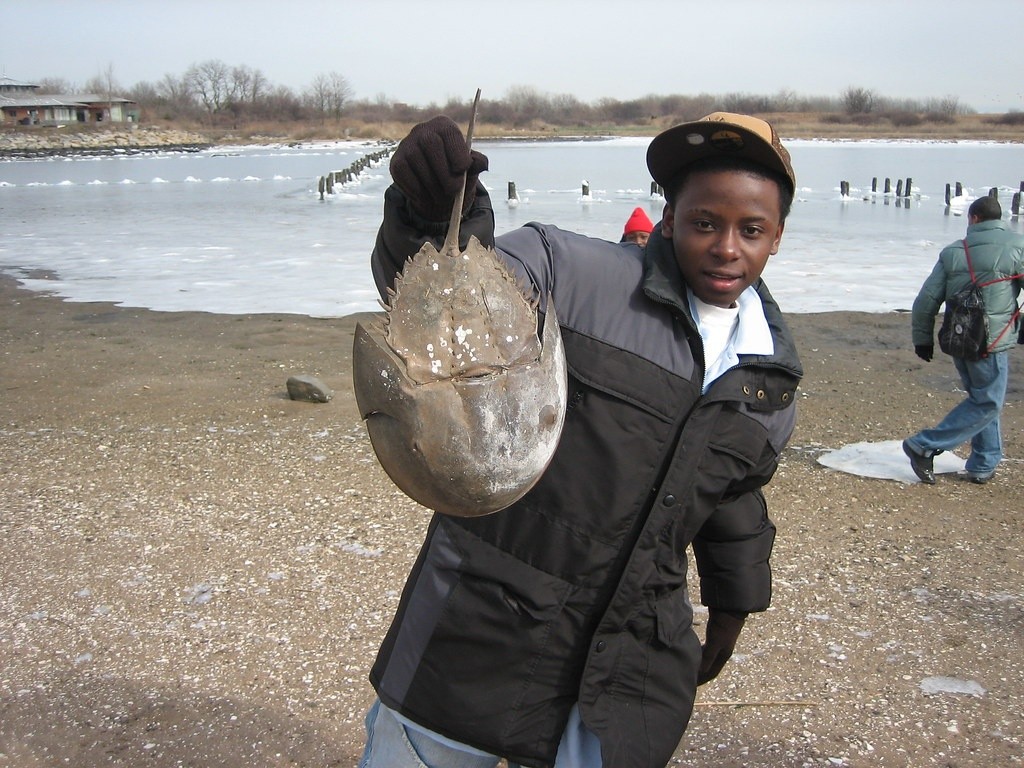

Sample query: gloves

[697,607,745,686]
[915,345,933,362]
[389,115,488,222]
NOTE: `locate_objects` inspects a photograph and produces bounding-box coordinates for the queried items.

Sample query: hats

[624,208,653,236]
[645,111,796,200]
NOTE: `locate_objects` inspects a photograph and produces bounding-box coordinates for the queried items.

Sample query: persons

[359,106,805,767]
[903,195,1024,487]
[620,206,656,247]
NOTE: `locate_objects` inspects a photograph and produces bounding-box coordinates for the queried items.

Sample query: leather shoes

[971,471,994,484]
[903,440,935,484]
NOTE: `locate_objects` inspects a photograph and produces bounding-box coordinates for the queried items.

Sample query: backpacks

[938,241,1024,360]
